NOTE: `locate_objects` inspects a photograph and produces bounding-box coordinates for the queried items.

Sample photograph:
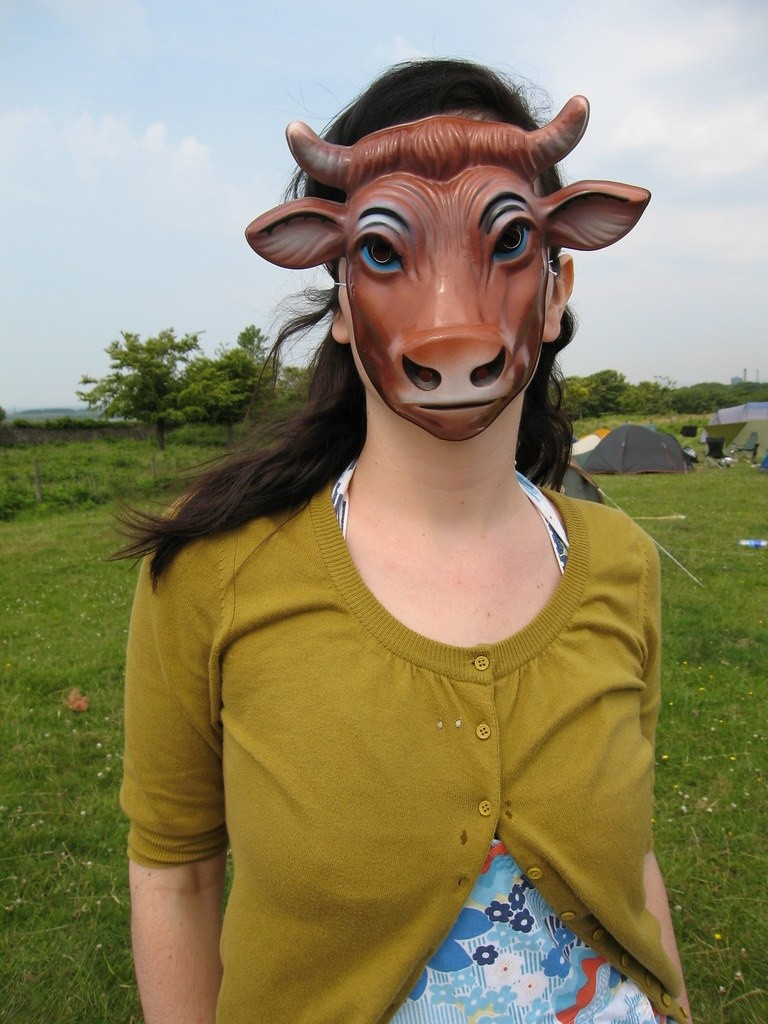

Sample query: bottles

[737,540,768,547]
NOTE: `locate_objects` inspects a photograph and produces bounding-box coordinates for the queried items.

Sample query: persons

[101,58,693,1024]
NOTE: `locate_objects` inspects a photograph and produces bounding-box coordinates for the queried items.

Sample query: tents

[572,423,694,474]
[700,402,768,443]
[559,463,604,504]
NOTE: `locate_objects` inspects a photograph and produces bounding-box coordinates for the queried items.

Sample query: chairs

[729,431,760,464]
[702,436,731,470]
